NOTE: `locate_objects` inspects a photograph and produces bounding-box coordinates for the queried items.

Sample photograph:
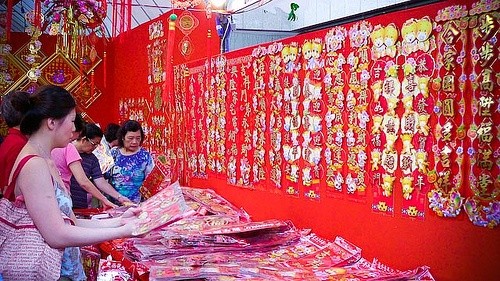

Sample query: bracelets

[100,196,106,203]
[117,194,122,201]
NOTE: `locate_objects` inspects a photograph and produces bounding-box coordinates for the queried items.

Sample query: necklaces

[29,138,62,176]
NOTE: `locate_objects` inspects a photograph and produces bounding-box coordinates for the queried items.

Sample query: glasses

[87,137,97,148]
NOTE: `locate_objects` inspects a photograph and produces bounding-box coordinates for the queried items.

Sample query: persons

[0,85,171,281]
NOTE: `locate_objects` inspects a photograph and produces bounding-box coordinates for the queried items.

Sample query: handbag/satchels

[0,155,76,281]
